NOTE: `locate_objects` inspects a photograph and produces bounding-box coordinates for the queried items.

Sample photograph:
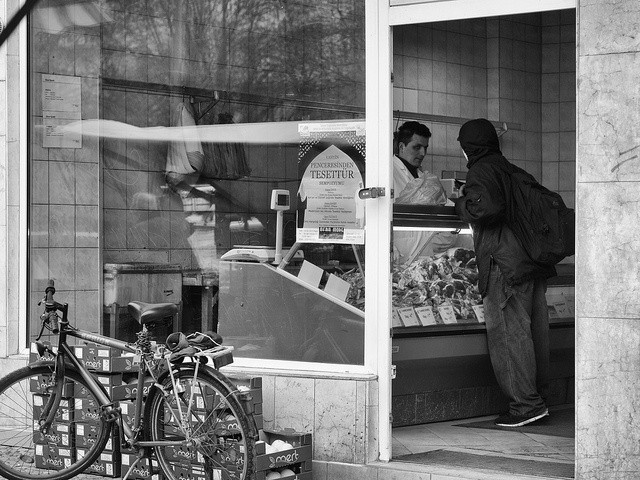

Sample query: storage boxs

[104,260,183,308]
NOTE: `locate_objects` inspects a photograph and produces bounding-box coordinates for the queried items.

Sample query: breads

[339,248,489,309]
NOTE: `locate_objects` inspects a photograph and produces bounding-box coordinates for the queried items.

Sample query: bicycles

[1,279,256,480]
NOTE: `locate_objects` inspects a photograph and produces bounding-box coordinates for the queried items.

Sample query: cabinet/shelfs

[219,209,575,426]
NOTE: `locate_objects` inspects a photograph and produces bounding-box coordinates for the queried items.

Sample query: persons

[450,119,556,428]
[392,121,447,206]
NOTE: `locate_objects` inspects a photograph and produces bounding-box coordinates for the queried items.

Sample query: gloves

[165,331,223,365]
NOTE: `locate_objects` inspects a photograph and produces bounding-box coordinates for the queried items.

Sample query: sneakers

[494,407,549,427]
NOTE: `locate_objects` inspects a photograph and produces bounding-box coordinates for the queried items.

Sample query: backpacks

[479,155,575,265]
